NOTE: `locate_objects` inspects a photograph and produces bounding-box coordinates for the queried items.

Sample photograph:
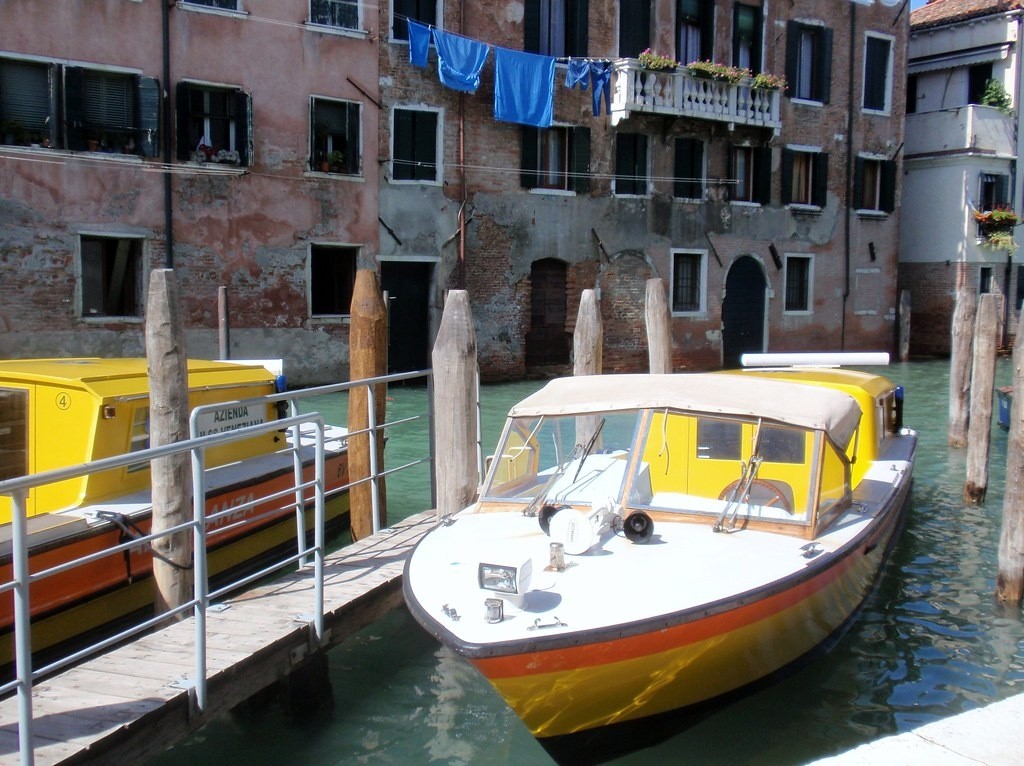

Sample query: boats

[402,353,917,740]
[0,356,389,670]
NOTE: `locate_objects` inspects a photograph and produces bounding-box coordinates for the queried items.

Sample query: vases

[985,218,1017,226]
[755,81,779,91]
[87,140,99,152]
[644,61,677,74]
[688,68,734,83]
[121,145,130,154]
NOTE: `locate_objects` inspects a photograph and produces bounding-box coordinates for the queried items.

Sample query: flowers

[188,148,241,165]
[638,48,681,70]
[749,70,790,92]
[687,59,753,87]
[987,207,1019,225]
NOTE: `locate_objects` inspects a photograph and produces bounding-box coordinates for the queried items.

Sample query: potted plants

[983,231,1020,258]
[0,119,50,148]
[320,150,346,172]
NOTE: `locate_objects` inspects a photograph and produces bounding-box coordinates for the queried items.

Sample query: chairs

[723,478,795,514]
